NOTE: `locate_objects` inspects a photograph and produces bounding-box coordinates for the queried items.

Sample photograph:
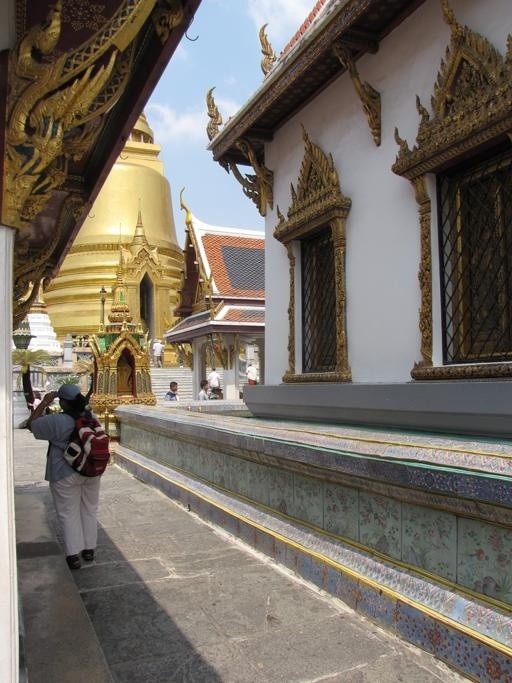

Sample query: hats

[51,384,81,400]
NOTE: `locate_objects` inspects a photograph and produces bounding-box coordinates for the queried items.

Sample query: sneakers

[81,549,94,561]
[67,556,81,569]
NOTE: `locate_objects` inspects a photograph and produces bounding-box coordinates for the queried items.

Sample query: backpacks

[61,411,109,477]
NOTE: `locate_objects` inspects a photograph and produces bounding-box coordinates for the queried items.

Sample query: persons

[26,380,111,571]
[151,337,164,367]
[245,362,258,384]
[164,380,178,401]
[208,366,220,390]
[199,379,211,401]
